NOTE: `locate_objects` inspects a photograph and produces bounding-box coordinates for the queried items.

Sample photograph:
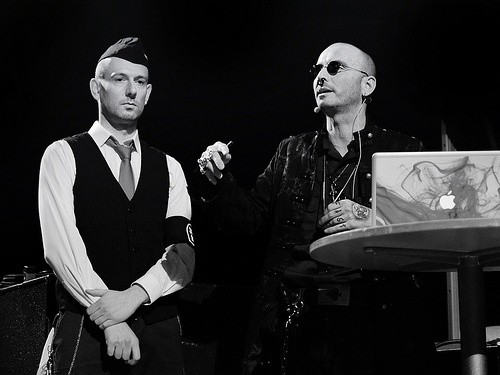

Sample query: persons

[198,40,424,375]
[35,36,195,375]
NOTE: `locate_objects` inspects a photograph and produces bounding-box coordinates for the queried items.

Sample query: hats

[98,37,149,69]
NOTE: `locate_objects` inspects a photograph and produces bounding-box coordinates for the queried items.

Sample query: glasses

[310,61,369,77]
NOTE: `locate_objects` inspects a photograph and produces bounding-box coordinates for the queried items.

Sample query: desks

[309,217,500,375]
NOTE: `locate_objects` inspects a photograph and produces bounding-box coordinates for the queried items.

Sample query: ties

[104,136,136,201]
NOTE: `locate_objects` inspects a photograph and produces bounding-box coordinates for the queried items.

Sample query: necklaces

[326,161,350,204]
